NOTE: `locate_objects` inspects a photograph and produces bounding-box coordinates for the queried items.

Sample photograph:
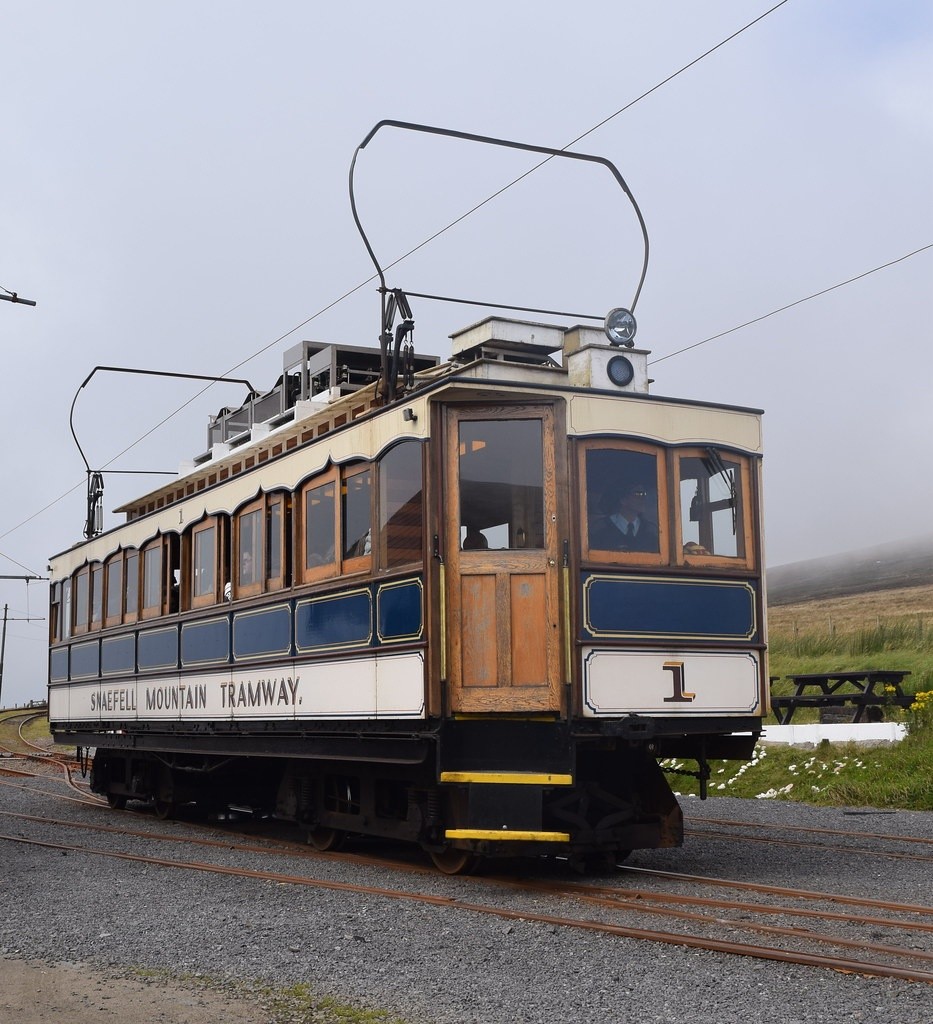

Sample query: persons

[588,480,659,554]
[75,521,369,629]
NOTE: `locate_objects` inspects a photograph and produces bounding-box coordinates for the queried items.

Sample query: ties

[626,521,636,538]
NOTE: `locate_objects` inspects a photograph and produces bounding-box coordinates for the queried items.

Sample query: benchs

[770,693,881,729]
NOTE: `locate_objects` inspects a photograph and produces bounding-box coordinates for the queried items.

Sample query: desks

[784,670,915,725]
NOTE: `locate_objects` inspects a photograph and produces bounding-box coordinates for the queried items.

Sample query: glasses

[625,490,649,498]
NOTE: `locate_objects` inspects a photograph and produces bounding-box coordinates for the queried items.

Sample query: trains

[46,120,768,873]
